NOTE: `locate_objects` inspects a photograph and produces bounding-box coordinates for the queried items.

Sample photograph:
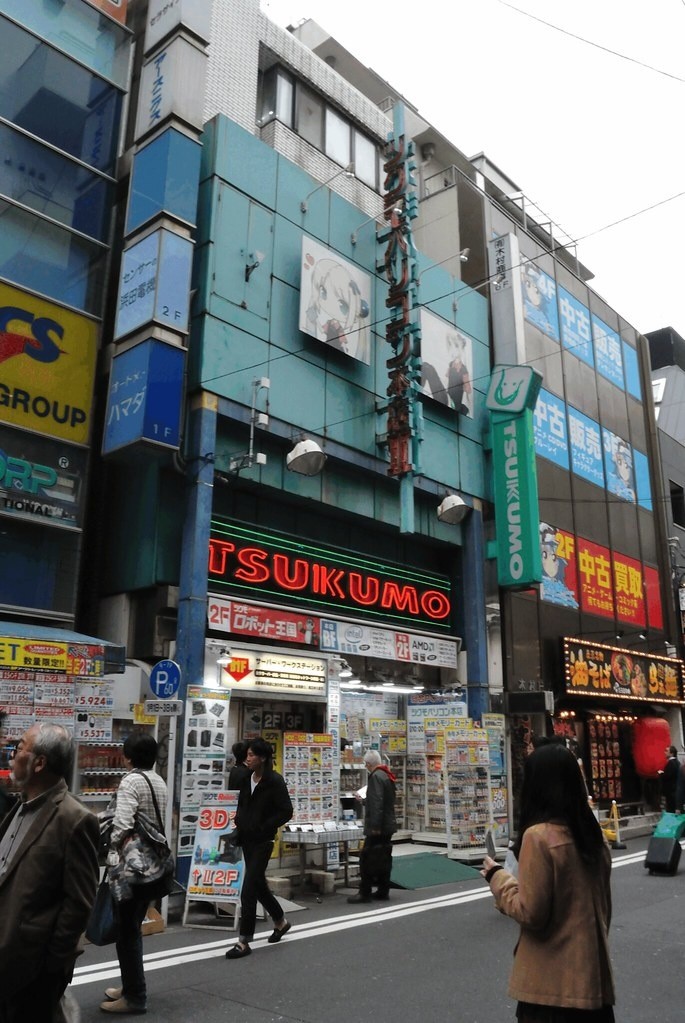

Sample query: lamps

[210,641,233,664]
[351,199,403,245]
[301,162,355,213]
[286,433,327,477]
[417,248,470,286]
[330,657,424,690]
[453,275,503,311]
[574,630,625,639]
[601,630,649,644]
[437,490,469,526]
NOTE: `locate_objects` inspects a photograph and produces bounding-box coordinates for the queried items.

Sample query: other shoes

[373,891,390,901]
[346,892,372,904]
[268,922,290,943]
[226,942,251,959]
[98,998,148,1013]
[105,987,123,998]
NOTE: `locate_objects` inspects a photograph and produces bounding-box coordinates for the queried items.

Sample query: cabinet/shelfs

[72,741,158,802]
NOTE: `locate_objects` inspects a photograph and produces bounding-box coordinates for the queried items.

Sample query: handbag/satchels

[86,883,125,947]
[655,812,684,838]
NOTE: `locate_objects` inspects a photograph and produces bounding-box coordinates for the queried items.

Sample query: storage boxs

[141,908,165,935]
[281,827,364,844]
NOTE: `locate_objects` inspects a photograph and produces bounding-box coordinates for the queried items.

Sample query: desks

[279,836,366,904]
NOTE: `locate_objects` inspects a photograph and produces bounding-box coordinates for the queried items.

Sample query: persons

[480,744,618,1023]
[512,735,585,808]
[0,711,100,1023]
[101,732,168,1013]
[659,746,685,814]
[226,739,294,959]
[347,750,397,904]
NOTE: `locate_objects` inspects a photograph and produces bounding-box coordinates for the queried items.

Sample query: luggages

[644,837,682,875]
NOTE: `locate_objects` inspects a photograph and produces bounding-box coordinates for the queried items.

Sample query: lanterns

[635,716,670,777]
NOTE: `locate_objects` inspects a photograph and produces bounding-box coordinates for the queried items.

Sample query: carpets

[256,895,307,917]
[388,850,483,889]
